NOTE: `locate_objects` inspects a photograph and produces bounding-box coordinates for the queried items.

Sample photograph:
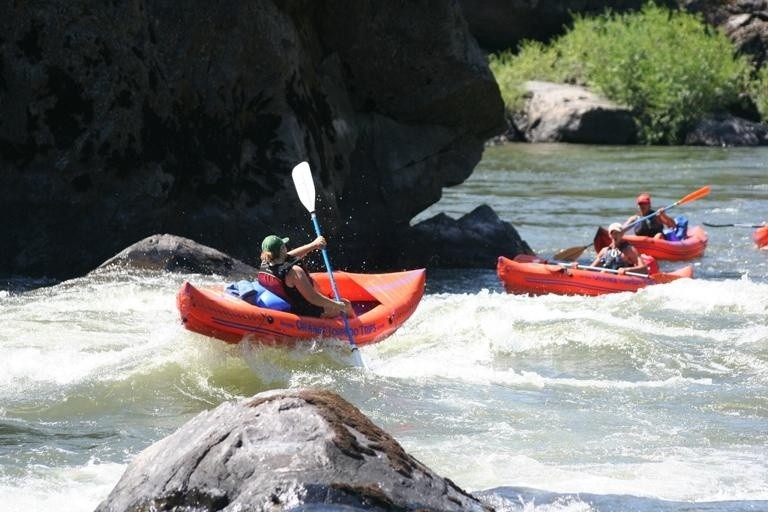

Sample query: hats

[609,223,622,233]
[262,235,289,251]
[637,194,650,203]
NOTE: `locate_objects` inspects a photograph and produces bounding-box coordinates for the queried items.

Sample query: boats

[181,266,429,349]
[495,253,692,296]
[593,223,706,262]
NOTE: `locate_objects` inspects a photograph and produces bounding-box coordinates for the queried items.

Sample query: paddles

[291,161,363,366]
[703,222,766,228]
[514,254,683,284]
[553,186,710,263]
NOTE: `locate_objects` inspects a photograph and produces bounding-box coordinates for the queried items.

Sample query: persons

[258,234,359,319]
[623,193,677,241]
[572,222,648,278]
